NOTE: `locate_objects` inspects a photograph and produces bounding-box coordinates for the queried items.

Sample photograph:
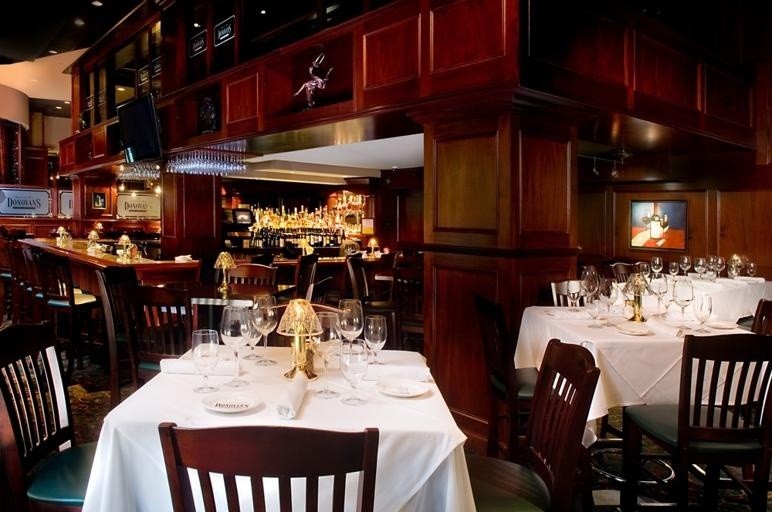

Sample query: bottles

[251,206,344,249]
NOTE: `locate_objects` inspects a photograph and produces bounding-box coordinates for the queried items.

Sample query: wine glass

[310,298,389,407]
[116,140,248,179]
[190,328,219,392]
[220,296,280,388]
[566,254,759,334]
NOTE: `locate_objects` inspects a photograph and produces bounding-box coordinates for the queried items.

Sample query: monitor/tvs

[116,90,164,165]
[232,208,254,226]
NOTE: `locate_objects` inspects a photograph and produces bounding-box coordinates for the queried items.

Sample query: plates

[202,390,264,413]
[376,377,432,398]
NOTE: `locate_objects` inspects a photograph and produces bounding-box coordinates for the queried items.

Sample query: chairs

[616,328,770,512]
[1,321,97,509]
[462,338,601,512]
[480,257,771,456]
[4,236,420,407]
[153,420,381,512]
